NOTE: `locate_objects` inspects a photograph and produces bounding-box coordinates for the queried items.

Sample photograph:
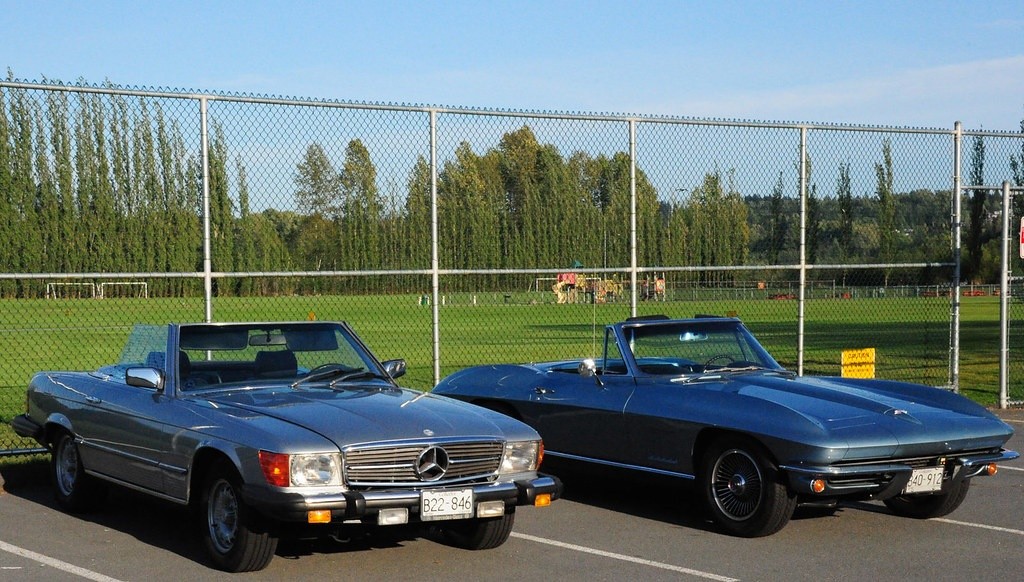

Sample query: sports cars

[427,313,1022,539]
[9,313,563,574]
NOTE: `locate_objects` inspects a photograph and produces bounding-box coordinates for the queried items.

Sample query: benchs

[558,359,724,375]
[183,359,253,382]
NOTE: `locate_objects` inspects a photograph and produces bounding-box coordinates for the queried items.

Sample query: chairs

[244,350,299,378]
[148,350,206,390]
